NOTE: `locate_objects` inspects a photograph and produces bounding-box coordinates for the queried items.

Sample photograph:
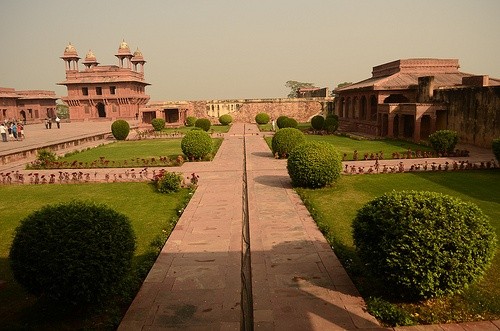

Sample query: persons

[0,116,60,143]
